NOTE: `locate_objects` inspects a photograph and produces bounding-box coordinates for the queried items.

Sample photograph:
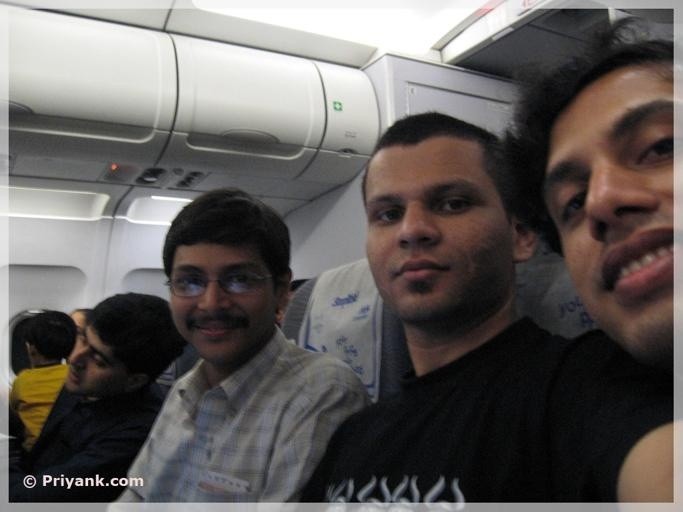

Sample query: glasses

[165,270,275,297]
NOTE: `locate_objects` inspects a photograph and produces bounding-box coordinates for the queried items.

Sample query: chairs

[159,242,595,403]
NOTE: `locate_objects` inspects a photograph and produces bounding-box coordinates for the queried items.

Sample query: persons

[7,291,187,502]
[7,310,77,462]
[62,307,97,368]
[100,182,377,502]
[476,14,673,504]
[292,106,619,503]
